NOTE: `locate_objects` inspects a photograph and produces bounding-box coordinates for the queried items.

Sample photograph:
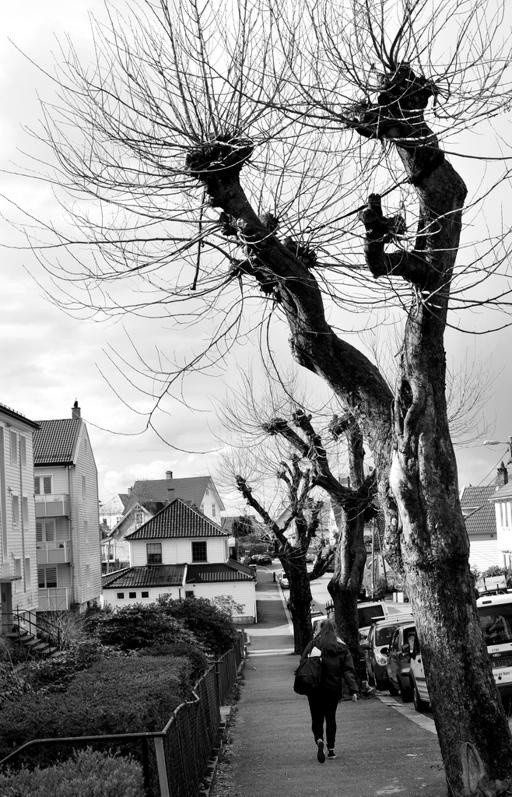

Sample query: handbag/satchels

[294,658,324,696]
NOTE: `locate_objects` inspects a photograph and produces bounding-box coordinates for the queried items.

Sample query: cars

[274,567,292,589]
[315,582,512,713]
[302,606,324,629]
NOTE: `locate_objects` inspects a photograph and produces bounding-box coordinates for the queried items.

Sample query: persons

[299,618,360,764]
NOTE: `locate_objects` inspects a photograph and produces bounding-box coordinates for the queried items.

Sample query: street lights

[484,437,512,455]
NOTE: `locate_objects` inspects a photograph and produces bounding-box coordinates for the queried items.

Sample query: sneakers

[328,749,336,759]
[317,741,325,763]
